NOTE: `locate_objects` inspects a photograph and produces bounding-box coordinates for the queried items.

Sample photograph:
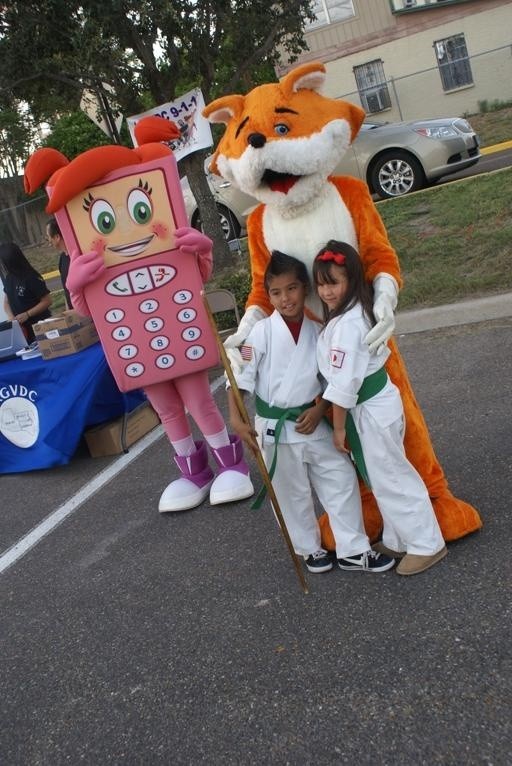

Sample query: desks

[0,342,145,475]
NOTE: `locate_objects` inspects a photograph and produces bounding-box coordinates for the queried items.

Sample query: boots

[210,435,253,505]
[158,440,215,512]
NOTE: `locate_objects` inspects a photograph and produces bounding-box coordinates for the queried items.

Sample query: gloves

[223,305,269,379]
[365,272,399,355]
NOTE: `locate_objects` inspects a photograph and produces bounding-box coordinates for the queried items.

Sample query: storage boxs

[33,309,99,362]
[83,405,161,457]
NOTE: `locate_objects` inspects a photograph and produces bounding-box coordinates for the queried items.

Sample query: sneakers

[396,547,448,575]
[370,542,406,559]
[304,548,333,573]
[339,550,395,573]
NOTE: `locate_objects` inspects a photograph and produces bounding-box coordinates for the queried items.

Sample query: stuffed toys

[25,115,253,514]
[200,62,484,553]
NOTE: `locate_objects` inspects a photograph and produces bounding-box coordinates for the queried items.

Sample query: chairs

[204,290,241,343]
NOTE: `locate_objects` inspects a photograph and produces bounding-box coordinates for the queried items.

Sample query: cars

[180,117,482,241]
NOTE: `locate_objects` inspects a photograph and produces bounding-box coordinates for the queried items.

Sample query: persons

[226,250,395,572]
[313,241,448,576]
[0,243,52,341]
[46,219,74,312]
